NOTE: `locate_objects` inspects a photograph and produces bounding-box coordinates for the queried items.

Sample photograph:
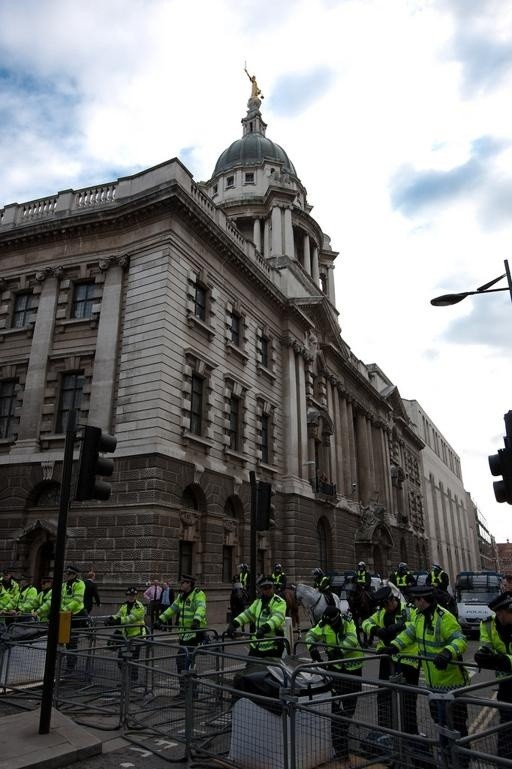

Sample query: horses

[341,575,377,650]
[378,579,407,603]
[230,574,254,633]
[435,592,460,621]
[291,582,342,628]
[255,572,302,640]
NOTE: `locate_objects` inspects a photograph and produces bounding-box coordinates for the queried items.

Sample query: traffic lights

[97,432,117,500]
[484,451,506,506]
[270,488,277,528]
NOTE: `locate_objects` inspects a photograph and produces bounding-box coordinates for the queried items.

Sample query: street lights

[427,260,512,314]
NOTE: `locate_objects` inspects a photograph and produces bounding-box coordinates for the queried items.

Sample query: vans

[328,572,503,637]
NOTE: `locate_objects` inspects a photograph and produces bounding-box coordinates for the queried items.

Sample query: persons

[306,607,364,764]
[501,574,511,593]
[142,578,180,632]
[154,575,207,699]
[362,585,471,769]
[231,563,459,649]
[474,591,512,769]
[104,587,145,689]
[226,578,286,669]
[0,561,102,681]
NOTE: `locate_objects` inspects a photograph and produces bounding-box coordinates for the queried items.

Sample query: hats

[375,586,393,604]
[257,577,274,590]
[39,575,53,584]
[66,565,79,575]
[488,590,511,612]
[319,607,342,628]
[126,587,138,596]
[409,585,434,601]
[180,574,197,583]
[19,575,33,581]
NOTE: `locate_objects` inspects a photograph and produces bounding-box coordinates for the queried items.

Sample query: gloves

[104,617,118,625]
[310,646,320,661]
[190,619,199,631]
[377,623,402,639]
[257,625,270,639]
[433,648,451,669]
[475,646,505,671]
[376,645,397,655]
[152,620,162,630]
[228,622,238,639]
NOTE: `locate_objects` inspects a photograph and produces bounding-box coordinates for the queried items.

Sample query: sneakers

[172,695,200,701]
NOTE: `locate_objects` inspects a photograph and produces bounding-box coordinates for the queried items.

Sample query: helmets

[275,563,282,572]
[397,562,407,570]
[2,569,13,575]
[432,562,441,570]
[313,566,325,576]
[238,562,247,571]
[358,560,366,569]
